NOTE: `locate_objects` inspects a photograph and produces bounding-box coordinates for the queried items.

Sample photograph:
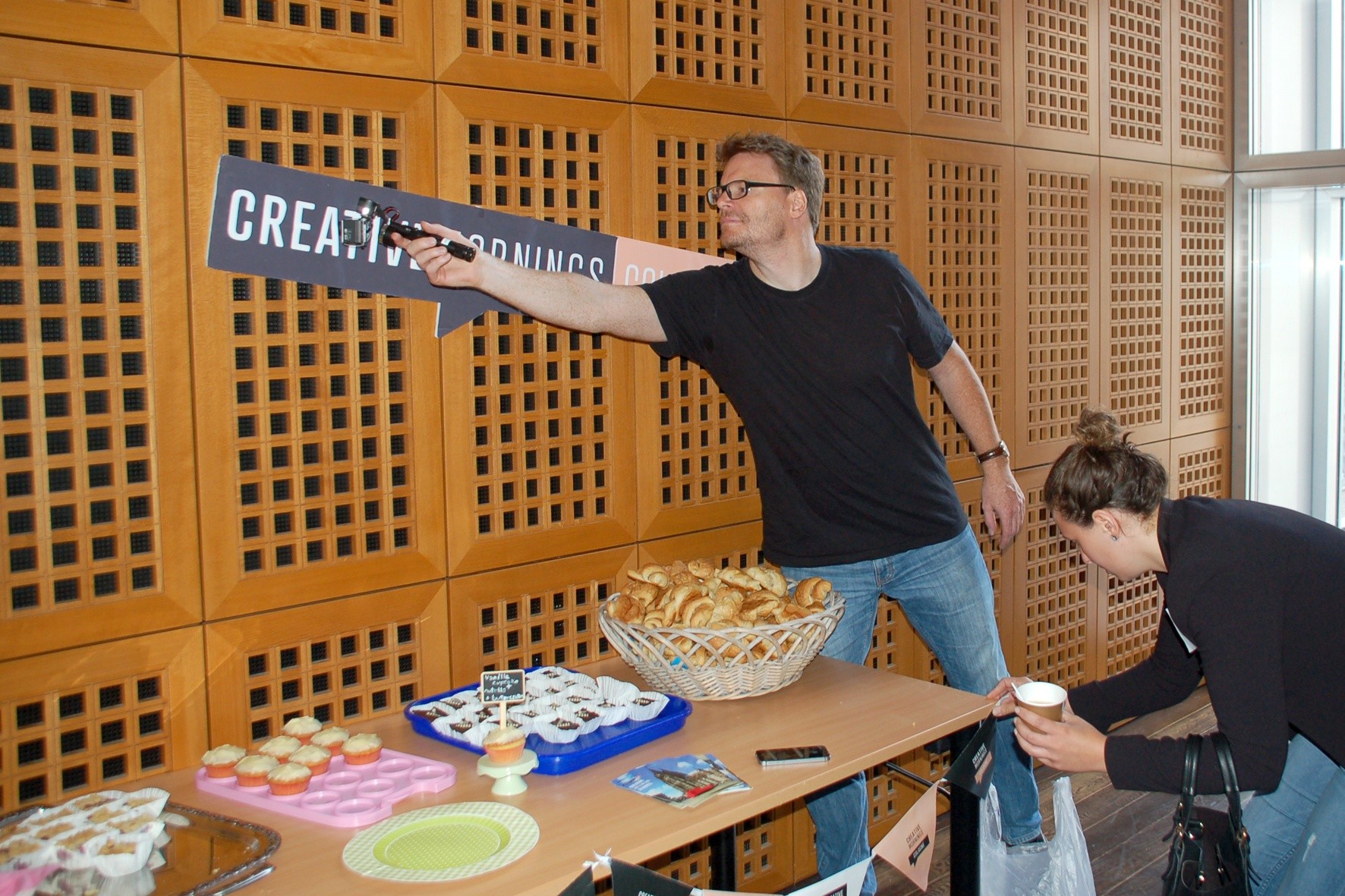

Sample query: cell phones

[756,745,831,766]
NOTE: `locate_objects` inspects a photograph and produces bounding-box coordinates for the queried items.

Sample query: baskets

[594,577,845,704]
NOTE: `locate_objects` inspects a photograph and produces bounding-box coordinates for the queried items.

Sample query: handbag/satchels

[976,774,1098,896]
[1161,731,1261,896]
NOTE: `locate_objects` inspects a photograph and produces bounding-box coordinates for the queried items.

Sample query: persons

[987,404,1345,896]
[391,127,1057,896]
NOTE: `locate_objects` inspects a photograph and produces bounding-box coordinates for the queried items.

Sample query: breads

[608,556,833,670]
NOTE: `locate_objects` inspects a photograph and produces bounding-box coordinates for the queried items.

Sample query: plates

[342,800,539,882]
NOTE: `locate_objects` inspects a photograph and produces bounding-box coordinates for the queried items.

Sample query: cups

[1016,681,1068,733]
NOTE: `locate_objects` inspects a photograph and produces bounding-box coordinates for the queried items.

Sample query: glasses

[706,181,795,207]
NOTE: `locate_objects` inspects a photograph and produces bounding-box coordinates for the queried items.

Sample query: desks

[117,652,998,896]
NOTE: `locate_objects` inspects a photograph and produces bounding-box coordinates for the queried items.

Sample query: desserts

[0,787,171,896]
[201,714,384,796]
[410,663,670,762]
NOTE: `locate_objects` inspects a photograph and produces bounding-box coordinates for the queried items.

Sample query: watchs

[973,439,1010,464]
